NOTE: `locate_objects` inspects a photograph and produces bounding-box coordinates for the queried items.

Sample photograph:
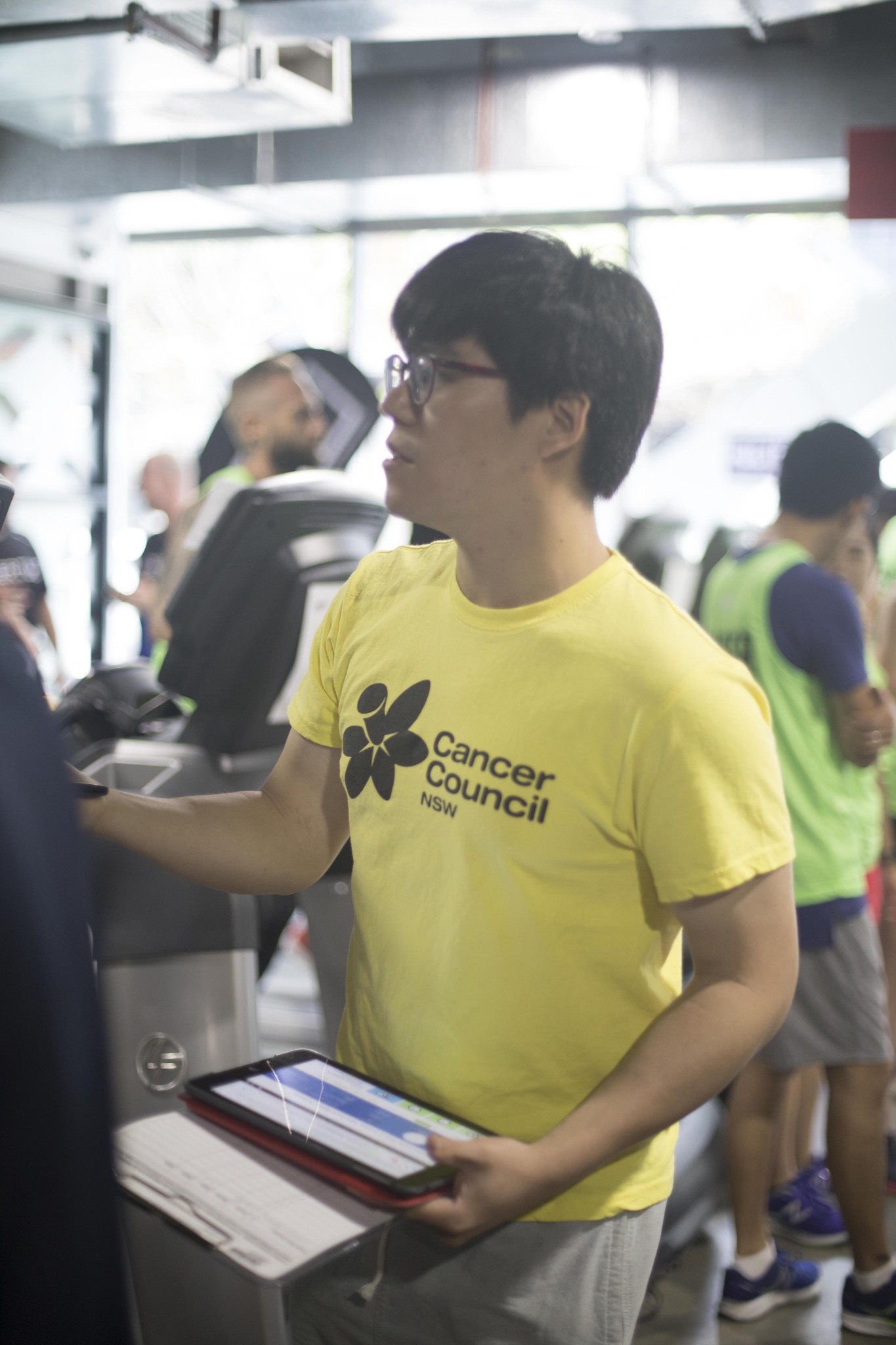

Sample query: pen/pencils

[71,784,107,800]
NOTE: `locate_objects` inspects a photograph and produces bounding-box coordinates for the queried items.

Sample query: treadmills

[619,512,763,629]
[59,467,395,1345]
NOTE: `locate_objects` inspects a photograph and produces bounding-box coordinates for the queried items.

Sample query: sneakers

[765,1164,850,1248]
[718,1251,821,1321]
[841,1272,895,1337]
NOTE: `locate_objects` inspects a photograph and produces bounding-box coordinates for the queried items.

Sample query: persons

[763,525,896,1247]
[104,457,189,662]
[0,525,58,652]
[150,362,330,715]
[64,231,796,1345]
[695,424,896,1336]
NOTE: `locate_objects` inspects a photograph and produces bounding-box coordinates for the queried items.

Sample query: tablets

[184,1049,500,1196]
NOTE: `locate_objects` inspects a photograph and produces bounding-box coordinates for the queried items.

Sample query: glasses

[385,352,507,407]
